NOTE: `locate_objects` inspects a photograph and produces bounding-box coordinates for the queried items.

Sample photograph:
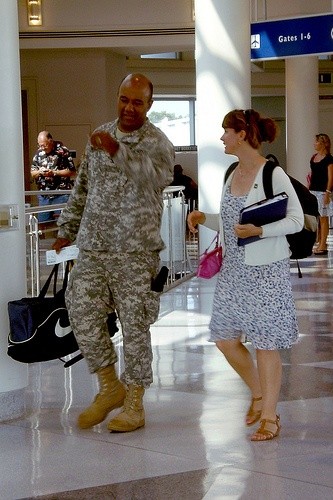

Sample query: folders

[237,192,289,246]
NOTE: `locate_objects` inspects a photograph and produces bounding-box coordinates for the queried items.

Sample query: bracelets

[326,189,331,193]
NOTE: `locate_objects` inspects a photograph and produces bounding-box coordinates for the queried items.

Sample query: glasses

[36,142,50,149]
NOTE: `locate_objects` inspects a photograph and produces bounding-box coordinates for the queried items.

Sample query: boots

[76,365,125,429]
[107,383,146,432]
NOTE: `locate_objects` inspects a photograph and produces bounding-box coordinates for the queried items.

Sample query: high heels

[245,396,262,426]
[251,415,281,441]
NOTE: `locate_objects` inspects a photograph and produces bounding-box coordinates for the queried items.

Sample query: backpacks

[224,154,319,259]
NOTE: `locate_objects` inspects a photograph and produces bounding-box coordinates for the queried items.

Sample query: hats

[174,164,183,171]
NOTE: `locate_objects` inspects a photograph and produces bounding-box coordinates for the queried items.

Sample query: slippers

[314,245,329,256]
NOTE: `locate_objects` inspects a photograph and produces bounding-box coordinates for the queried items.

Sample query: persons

[170,165,198,238]
[30,131,75,239]
[52,71,175,432]
[308,134,333,255]
[187,109,305,441]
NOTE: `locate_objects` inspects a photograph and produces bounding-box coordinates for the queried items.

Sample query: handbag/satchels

[6,259,119,367]
[195,232,223,279]
